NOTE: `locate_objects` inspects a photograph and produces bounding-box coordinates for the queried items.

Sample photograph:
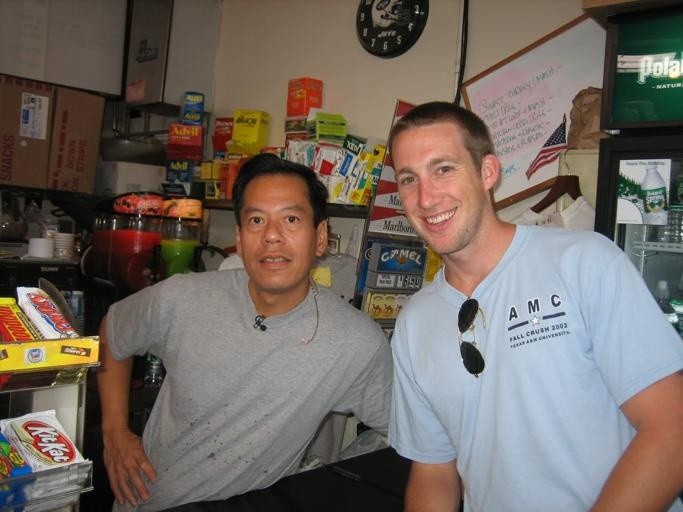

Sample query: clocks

[355,0,429,59]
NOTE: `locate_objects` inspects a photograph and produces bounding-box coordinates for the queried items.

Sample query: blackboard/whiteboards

[463,14,608,208]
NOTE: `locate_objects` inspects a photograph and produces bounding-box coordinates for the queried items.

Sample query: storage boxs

[48,84,106,196]
[0,73,55,190]
[95,159,168,198]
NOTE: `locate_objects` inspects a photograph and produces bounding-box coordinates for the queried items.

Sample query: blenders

[86,138,206,407]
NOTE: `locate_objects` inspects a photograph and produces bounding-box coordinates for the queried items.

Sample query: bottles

[653,274,683,336]
[641,162,668,225]
[138,360,164,427]
[655,173,682,243]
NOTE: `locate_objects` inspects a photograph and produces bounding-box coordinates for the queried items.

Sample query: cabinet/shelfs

[0,336,101,512]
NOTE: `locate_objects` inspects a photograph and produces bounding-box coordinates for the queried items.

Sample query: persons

[97,153,392,511]
[387,102,683,512]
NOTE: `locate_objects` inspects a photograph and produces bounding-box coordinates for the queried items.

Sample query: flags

[525,122,568,180]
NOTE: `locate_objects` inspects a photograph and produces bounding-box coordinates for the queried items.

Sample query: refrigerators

[592,8,683,347]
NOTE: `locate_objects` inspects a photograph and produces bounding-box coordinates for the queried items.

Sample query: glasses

[459,297,486,378]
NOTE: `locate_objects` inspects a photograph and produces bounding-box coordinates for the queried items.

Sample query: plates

[51,232,76,262]
